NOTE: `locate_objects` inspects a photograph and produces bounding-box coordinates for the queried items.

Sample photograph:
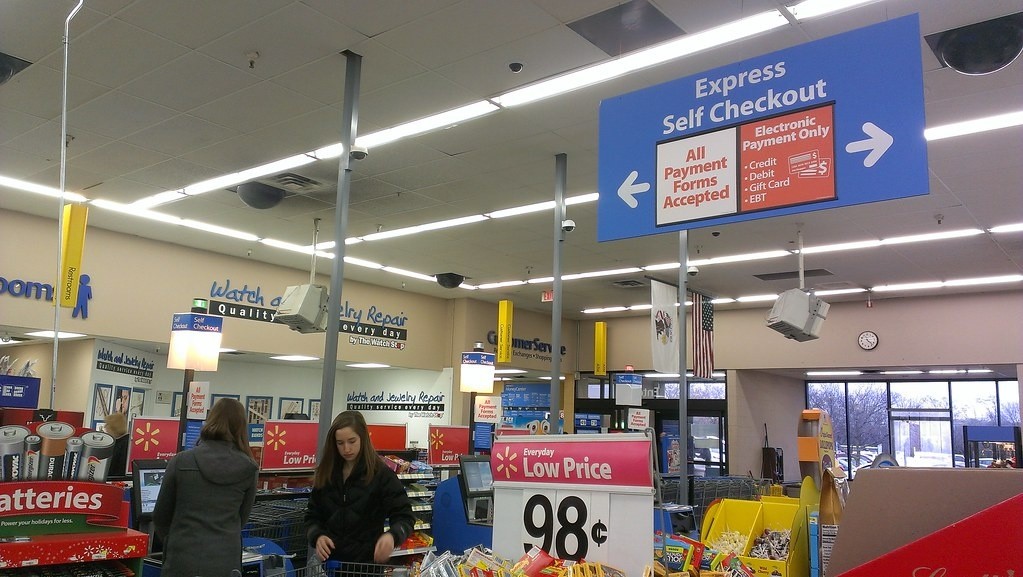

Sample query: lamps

[459,343,495,455]
[166,300,224,454]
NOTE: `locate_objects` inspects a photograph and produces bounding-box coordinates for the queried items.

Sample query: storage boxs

[702,499,809,577]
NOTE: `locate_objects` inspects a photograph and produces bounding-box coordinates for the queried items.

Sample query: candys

[750,527,791,561]
[709,529,748,556]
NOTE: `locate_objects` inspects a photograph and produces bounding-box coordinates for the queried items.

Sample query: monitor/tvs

[132,458,170,522]
[274,284,330,334]
[458,454,493,498]
[766,288,830,342]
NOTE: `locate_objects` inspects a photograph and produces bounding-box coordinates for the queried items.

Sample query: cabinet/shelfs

[384,473,438,557]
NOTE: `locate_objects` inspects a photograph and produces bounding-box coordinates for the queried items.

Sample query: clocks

[858,330,879,350]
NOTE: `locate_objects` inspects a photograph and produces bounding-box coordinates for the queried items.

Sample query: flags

[692,292,714,379]
[651,280,679,374]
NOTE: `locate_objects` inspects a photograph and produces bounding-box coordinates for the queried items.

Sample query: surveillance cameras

[350,145,368,160]
[508,64,523,73]
[712,231,720,237]
[940,13,1023,76]
[436,273,464,289]
[237,182,284,210]
[563,220,576,232]
[0,57,15,85]
[688,266,699,277]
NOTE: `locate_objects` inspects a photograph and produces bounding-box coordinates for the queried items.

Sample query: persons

[304,410,417,577]
[152,398,260,577]
[105,413,130,476]
[115,390,129,414]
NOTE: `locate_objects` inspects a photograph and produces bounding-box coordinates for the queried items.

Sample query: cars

[954,453,993,470]
[837,452,876,480]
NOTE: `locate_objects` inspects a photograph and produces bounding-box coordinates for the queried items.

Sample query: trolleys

[656,475,773,533]
[242,498,316,561]
[266,560,406,577]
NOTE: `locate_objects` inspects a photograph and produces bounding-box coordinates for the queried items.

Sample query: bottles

[409,441,419,448]
